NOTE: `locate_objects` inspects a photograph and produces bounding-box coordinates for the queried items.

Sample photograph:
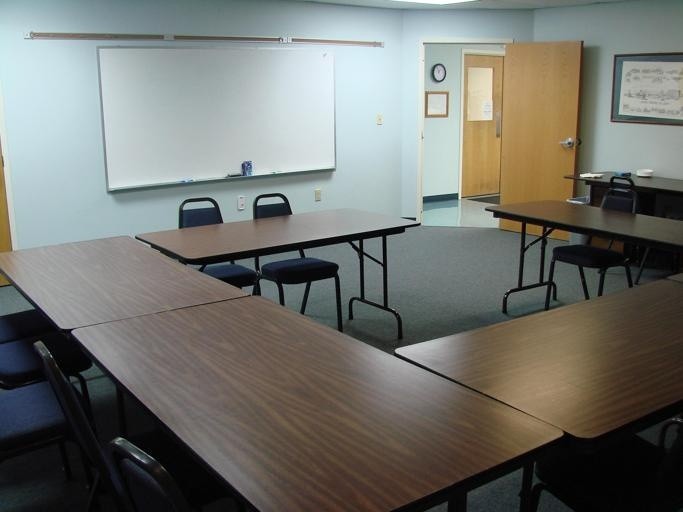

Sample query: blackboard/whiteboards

[97,45,338,193]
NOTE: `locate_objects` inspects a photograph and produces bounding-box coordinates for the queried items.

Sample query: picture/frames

[610,51,682,128]
[425,91,449,118]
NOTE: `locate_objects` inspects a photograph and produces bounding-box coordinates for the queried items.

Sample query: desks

[0,236,251,366]
[129,204,421,344]
[574,168,682,271]
[69,289,564,511]
[485,194,683,315]
[389,275,681,512]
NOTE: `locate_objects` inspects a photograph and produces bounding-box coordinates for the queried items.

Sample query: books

[579,172,605,178]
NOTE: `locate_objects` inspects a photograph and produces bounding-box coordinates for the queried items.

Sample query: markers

[181,180,193,182]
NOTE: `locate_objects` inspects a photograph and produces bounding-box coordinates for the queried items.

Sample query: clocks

[431,63,446,82]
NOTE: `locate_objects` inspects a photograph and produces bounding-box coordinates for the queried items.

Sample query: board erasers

[228,173,241,177]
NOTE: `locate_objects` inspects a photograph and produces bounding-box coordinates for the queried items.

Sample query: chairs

[108,429,205,509]
[525,391,682,512]
[248,190,347,334]
[0,304,127,474]
[34,341,137,510]
[174,189,262,303]
[539,173,642,310]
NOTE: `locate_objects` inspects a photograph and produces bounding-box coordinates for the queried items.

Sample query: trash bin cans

[566,196,590,246]
